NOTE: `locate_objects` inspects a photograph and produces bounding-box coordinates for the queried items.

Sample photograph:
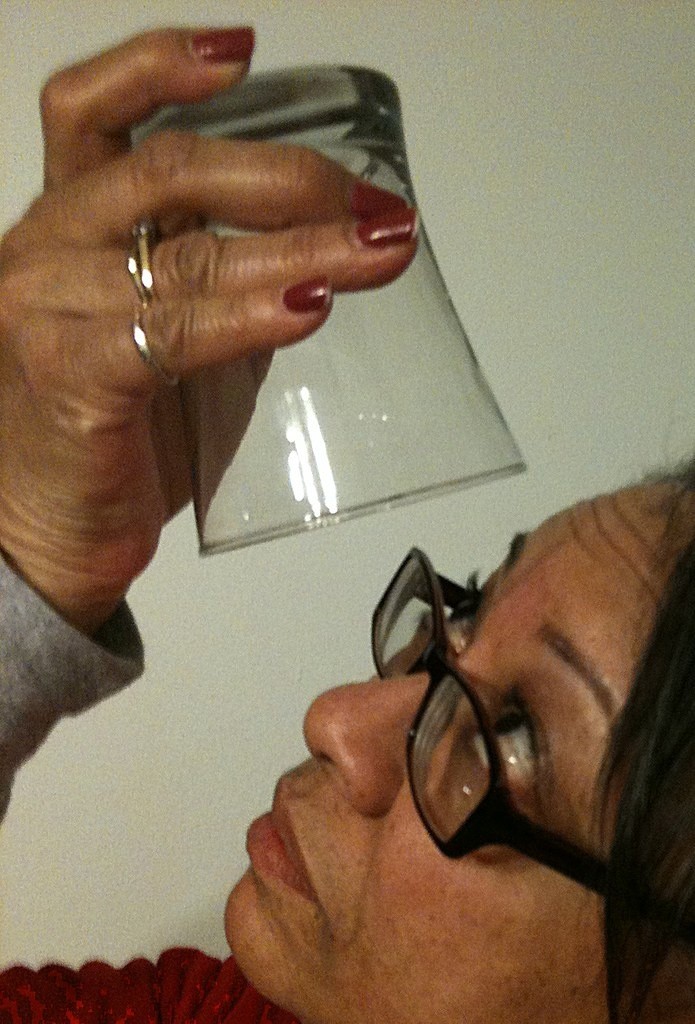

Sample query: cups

[131,66,529,559]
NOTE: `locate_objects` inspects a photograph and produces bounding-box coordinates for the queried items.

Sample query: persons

[0,27,695,1024]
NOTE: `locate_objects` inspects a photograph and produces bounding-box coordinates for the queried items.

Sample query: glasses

[372,546,608,898]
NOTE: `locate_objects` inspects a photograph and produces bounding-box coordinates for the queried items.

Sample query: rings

[129,223,161,305]
[133,305,187,389]
